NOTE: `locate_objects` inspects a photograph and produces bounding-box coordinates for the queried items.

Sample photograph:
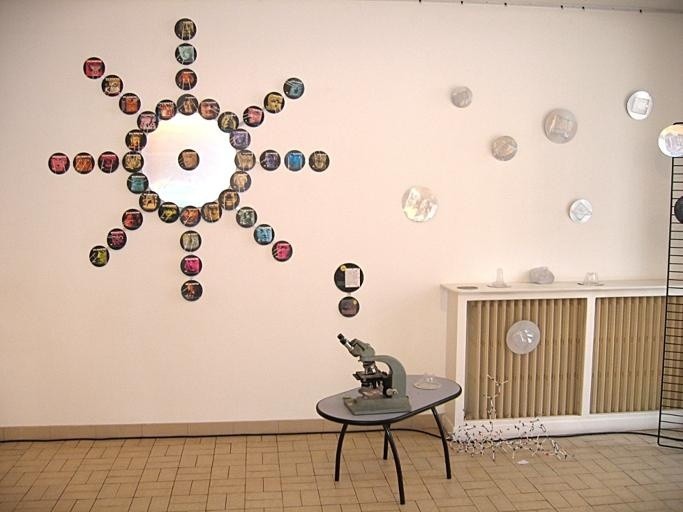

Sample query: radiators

[444,281,683,443]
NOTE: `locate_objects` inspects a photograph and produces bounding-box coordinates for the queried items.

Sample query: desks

[316,373,462,505]
